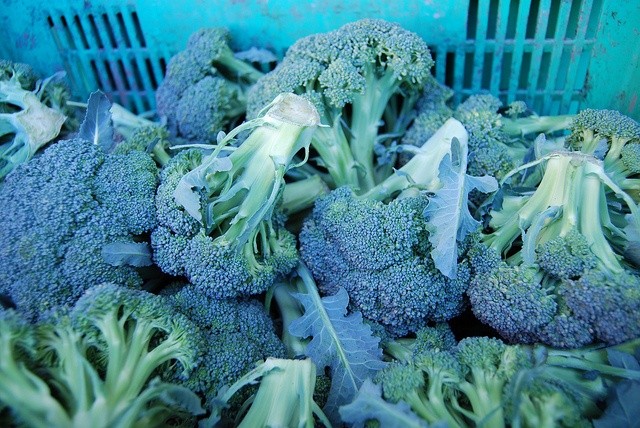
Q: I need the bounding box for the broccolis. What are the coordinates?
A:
[0,18,640,428]
